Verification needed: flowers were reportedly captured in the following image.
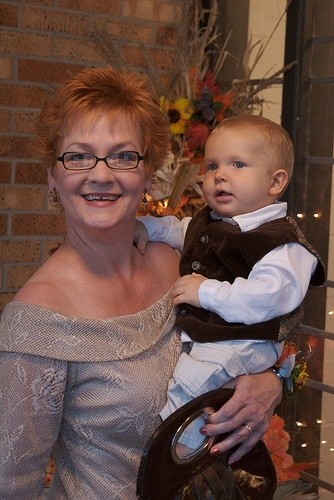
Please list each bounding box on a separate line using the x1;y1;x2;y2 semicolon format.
277;339;315;392
98;3;267;225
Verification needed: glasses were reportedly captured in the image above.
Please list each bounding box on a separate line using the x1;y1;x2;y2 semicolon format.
55;150;146;171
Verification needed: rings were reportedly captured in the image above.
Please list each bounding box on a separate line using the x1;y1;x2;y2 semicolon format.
242;424;253;435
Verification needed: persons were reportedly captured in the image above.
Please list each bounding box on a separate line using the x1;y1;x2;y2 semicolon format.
132;115;328;463
0;65;308;500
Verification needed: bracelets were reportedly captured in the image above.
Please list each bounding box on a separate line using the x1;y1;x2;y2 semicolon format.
269;341;314;396
